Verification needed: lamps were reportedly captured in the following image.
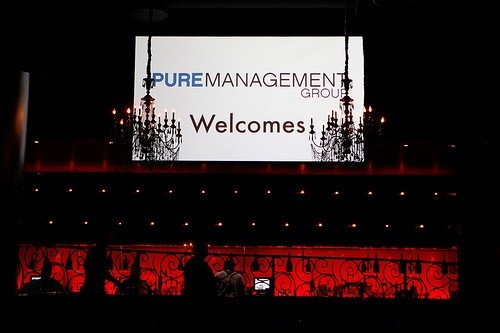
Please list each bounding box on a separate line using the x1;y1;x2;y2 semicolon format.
306;1;388;176
108;1;183;171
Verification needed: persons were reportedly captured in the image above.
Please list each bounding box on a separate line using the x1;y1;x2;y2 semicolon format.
121;265;153;296
214;259;245;297
41;261;64;287
84;236;121;296
183;237;219;297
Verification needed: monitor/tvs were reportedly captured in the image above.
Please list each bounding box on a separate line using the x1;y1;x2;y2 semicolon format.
30;275;41;286
253;276;272;291
